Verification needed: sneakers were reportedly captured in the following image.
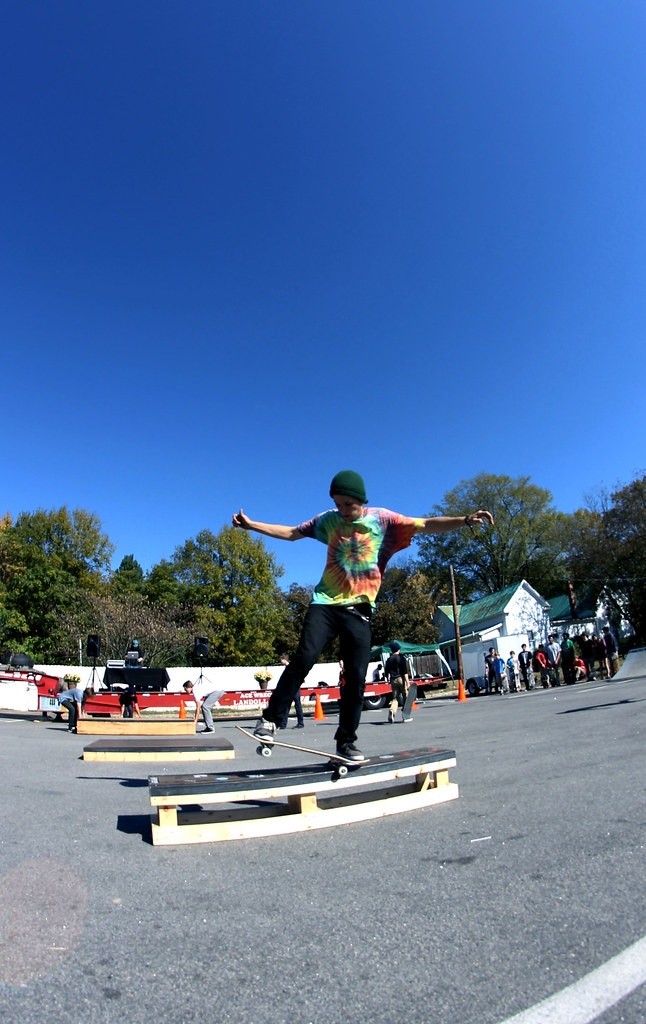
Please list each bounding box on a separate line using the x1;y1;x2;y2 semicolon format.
254;716;275;742
336;739;365;760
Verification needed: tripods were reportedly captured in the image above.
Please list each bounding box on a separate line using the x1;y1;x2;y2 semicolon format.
86;657;103;690
193;660;212;686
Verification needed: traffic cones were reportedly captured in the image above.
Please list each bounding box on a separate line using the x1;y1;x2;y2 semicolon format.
312;693;327;720
455;679;469;701
179;700;187;719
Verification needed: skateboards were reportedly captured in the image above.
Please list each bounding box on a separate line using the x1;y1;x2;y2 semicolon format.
232;723;371;776
514;669;522;691
528;667;536;690
575;678;588;684
546;665;556;687
402;683;418;720
501;671;509;695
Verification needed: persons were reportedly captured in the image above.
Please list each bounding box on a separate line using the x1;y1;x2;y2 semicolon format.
233;470;494;760
59;688;95;732
386;644;413;723
373;664;382;682
281;657;304;729
338;660;345;687
484;627;619;695
183;680;224;734
119;688;142;718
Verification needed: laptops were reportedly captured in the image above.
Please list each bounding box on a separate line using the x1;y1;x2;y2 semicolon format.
128;652;139;659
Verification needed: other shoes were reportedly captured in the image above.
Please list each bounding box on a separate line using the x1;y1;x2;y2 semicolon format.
69;727;75;734
200;729;214;734
403;717;413;722
388;710;395;724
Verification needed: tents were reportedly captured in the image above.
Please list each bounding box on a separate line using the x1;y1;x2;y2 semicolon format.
371;640;454;685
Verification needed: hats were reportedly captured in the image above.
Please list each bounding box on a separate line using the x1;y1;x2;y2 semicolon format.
388;642;400;653
330;471;366;504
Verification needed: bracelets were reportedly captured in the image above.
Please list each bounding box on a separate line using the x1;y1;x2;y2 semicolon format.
465;515;471;527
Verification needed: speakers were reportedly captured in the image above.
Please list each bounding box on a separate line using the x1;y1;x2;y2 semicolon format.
86;634;101;657
194;636;209;659
